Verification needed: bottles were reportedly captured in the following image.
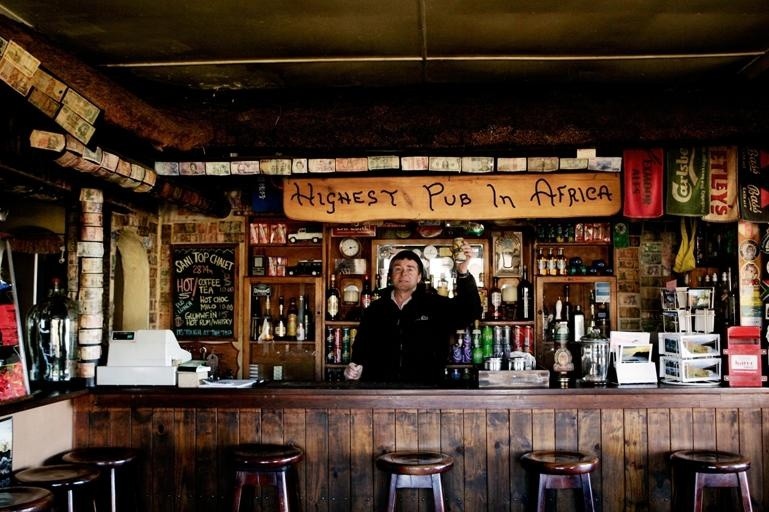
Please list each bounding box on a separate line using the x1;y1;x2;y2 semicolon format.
244;222;740;386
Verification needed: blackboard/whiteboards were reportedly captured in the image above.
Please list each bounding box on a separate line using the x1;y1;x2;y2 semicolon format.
170;243;240;341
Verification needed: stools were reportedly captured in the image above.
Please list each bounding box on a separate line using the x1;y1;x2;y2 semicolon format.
374;450;452;512
0;440;126;511
669;448;754;511
224;441;302;511
518;448;600;511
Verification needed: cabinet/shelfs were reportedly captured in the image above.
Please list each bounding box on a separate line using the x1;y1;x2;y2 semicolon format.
241;217;618;382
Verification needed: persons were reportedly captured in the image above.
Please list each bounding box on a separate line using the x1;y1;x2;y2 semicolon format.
343;242;483;387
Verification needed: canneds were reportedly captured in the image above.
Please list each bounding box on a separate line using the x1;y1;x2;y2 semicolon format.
453;326;536;363
453;237;466;264
326;329;357;365
